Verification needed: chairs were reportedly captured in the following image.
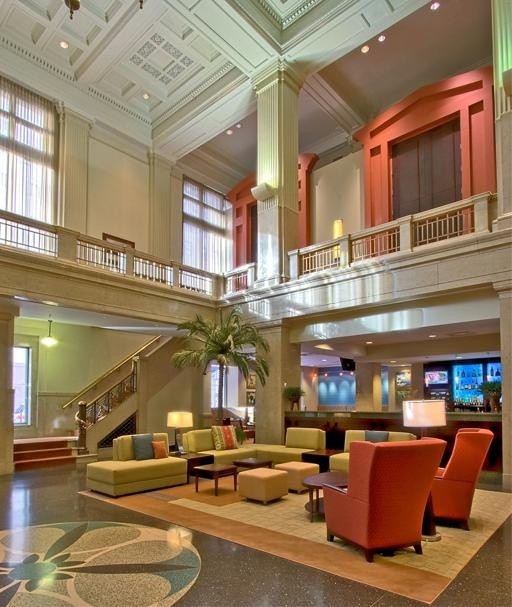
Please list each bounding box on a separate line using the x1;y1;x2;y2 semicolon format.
321;436;448;563
430;428;495;535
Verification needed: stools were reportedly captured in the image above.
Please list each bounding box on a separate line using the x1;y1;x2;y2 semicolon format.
274;460;320;494
236;467;289;504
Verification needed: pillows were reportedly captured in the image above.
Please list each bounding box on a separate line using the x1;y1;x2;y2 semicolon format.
131;432;169;461
211;425;239;450
365;430;390;442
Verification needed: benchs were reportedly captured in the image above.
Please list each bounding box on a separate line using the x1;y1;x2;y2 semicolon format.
257;426;326;469
183;429;283;473
86;431;189;498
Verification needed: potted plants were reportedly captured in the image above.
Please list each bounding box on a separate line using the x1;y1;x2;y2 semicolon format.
477;380;501;413
283;388;306;411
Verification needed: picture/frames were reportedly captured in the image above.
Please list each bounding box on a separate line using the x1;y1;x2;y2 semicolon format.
102;230;136;269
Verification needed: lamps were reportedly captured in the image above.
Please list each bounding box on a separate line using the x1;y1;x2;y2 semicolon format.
402;399;447;440
428;0;440;11
40;313;58;348
167;410;193;454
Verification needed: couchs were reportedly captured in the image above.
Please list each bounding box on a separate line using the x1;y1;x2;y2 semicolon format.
328;428;417;473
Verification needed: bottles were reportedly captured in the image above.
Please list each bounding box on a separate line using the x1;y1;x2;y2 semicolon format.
491;366;501;375
456;367;483;405
